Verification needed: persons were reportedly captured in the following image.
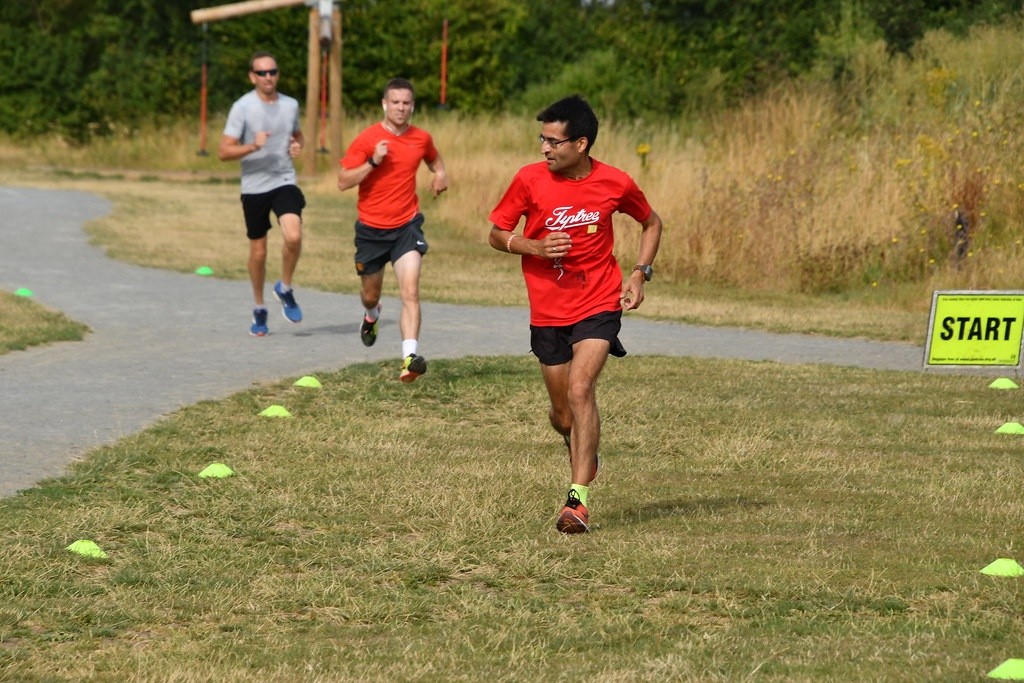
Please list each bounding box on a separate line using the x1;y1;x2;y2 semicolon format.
486;92;662;534
217;53;305;336
335;78;446;384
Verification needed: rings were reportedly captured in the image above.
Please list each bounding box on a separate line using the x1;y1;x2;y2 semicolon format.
553;247;556;252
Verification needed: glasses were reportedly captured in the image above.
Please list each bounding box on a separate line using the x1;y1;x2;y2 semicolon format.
252;68;277;76
539;132;581;148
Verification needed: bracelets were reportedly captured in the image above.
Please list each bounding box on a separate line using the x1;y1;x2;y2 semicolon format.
368;156;379;168
507;235;516;253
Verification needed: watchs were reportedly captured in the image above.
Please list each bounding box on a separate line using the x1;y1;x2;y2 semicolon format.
632;264;653;281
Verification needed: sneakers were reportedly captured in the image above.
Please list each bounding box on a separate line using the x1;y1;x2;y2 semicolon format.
250;304;270;337
400;354;426;384
274;281;303;324
555;488;588;533
359;302;383;347
